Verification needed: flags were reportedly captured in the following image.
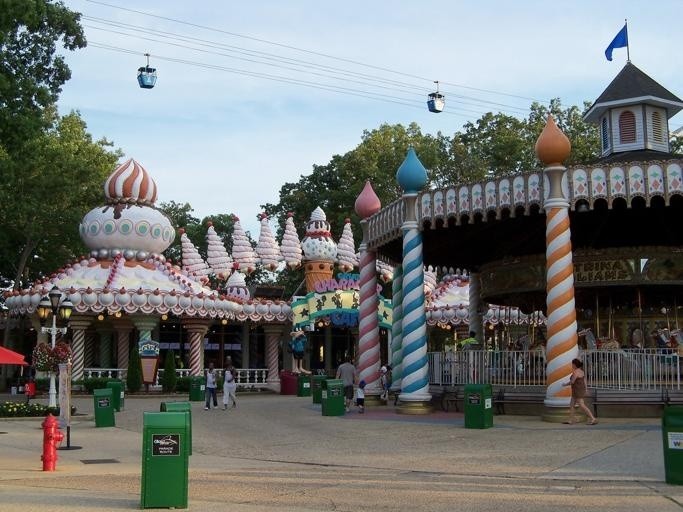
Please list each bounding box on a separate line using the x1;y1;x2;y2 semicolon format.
605;24;628;61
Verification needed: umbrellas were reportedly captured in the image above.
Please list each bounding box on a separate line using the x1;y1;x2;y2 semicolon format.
0;347;29;366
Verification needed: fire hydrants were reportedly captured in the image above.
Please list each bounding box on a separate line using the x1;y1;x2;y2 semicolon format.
38;413;64;470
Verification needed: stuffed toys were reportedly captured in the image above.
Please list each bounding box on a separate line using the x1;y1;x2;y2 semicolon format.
288;322;312;375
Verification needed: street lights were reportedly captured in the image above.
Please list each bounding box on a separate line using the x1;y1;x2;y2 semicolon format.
36;285;73;407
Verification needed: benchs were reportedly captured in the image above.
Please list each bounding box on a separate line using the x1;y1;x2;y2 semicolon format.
445;386;500;413
391;385;445;411
496;388;545;414
663;389;683;406
0;393;29;403
591;387;666;418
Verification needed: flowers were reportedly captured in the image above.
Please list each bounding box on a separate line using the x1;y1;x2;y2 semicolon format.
31;342;74;371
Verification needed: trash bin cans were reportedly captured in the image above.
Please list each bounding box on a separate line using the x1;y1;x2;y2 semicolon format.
313;375;327;403
465;384;493;429
662;406;683;485
322;379;344;416
189;377;205;401
107;382;124;412
141;402;192;509
297;376;311;397
93;388;115;427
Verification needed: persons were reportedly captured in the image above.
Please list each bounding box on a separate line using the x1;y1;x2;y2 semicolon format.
355;381;367;413
174;354;182;376
351;357;356;367
204;361;219;410
561;359;599;425
456;331;481;349
335;355;358;413
380;360;393;402
184;351;190;369
336;357;342;369
221;358;238;411
